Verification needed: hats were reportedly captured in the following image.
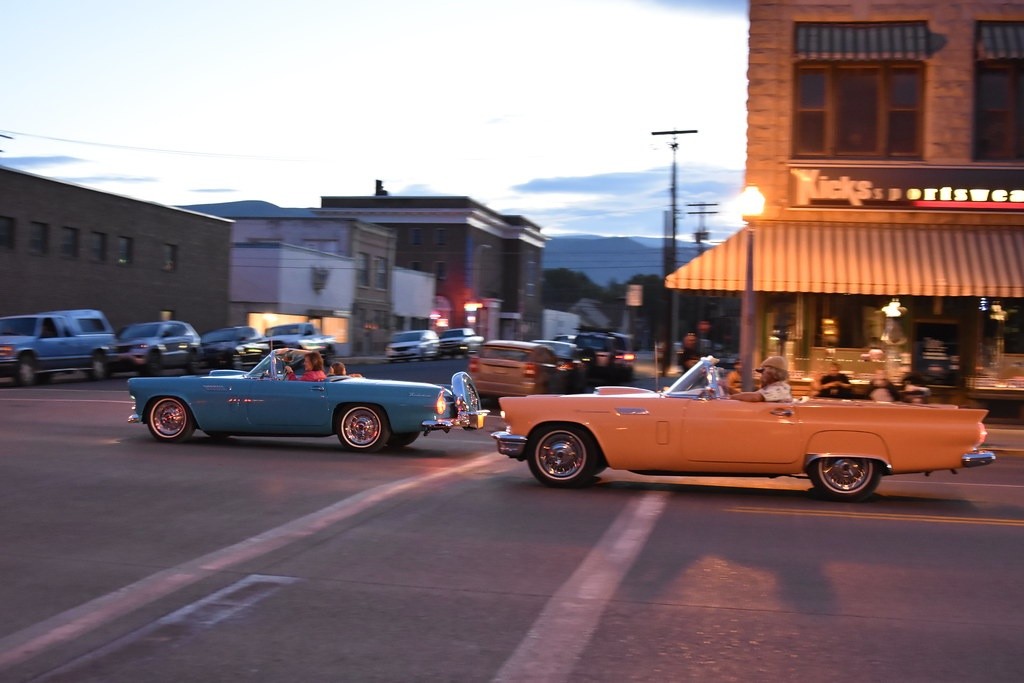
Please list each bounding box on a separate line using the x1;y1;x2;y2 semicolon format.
872;369;888;380
760;355;788;371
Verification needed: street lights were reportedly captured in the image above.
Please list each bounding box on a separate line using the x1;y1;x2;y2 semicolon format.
651;129;700;377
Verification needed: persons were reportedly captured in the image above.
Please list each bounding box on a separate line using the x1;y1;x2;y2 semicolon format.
694;318;715;357
717;355;793;403
864;369;901;403
329;362;346;375
816;362;853;399
283;351;327;382
678;331;701;376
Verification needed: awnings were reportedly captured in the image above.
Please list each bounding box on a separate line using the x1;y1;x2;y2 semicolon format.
666;223;1024;298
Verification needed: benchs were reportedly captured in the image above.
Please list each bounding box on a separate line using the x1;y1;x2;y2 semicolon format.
319;376;350;382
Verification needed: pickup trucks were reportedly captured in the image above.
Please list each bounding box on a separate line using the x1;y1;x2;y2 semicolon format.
1;309;120;388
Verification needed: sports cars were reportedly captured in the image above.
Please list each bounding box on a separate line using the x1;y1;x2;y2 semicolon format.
491;356;996;502
126;348;492;452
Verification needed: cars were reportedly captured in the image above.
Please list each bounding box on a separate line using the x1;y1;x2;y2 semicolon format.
469;341;574;401
554;331;639;381
263;324;336;367
111;321;204;377
436;327;485;358
385;329;441;363
200;326;270;370
533;339;591;394
